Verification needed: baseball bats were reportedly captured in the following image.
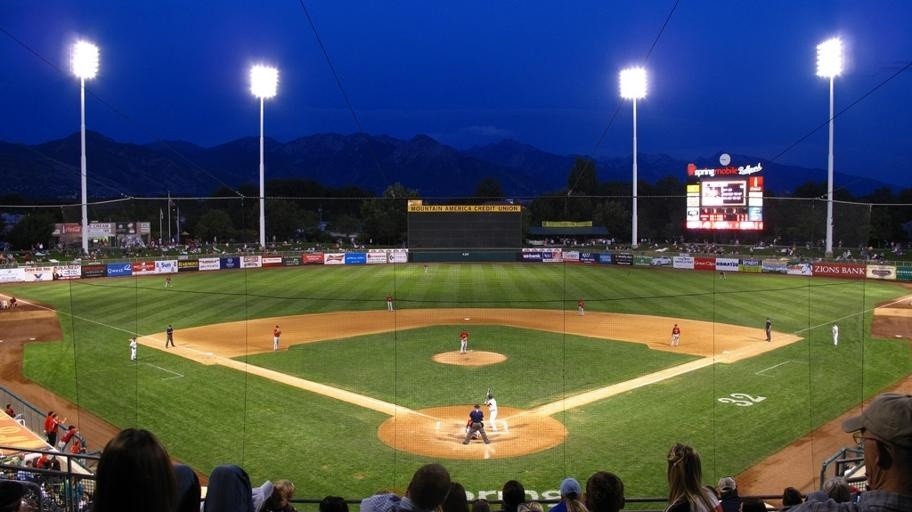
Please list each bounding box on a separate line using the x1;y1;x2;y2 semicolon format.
483;388;490;405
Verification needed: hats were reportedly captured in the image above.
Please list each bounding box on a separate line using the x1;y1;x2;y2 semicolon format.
842;392;912;447
718;477;737;493
824;477;850;502
252;480;273;512
561;478;580;499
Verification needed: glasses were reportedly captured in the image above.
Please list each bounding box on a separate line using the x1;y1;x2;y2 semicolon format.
853;432;889;448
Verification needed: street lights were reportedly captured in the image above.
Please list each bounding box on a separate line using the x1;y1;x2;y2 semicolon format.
248;62;280;252
619;65;650;251
69;40;102;256
816;35;850;263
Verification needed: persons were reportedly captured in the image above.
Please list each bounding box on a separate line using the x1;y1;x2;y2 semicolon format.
831;322;838;346
459;329;469;354
665;443;767;511
578;295;585;316
319;496;349;511
9;296;16;311
385;294;394;312
719;270;727;280
273;325;281;352
782;476;862;508
165;275;173;288
44;411;81;457
543;235;912;266
84;427;295;511
0;238;367;269
129;337;137;361
462;404;490;444
2;446;84;511
358;464;626;512
6;404;16;418
485;395;498;432
165;324;176;349
52;267;62;281
797;396;912;510
764;317;771;342
671;324;681;347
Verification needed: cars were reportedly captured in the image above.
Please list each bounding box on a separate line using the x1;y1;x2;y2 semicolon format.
650;255;671;267
